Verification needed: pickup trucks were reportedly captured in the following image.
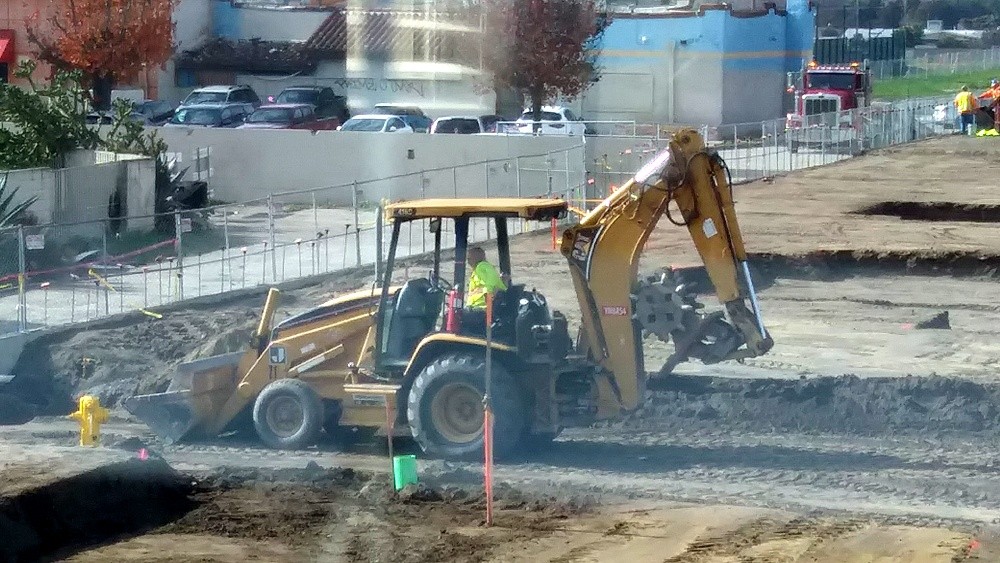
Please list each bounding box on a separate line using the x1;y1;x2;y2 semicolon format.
233;102;338;131
274;84;348;118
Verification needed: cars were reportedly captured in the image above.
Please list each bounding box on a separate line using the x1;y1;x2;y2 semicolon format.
127;98;175;127
84;110;157;127
429;115;509;134
515;105;588;135
369;102;434;134
163;100;248;128
336;113;414;132
932;105;960;129
173;84;262;124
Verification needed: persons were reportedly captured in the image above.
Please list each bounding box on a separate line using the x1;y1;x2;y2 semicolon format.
955;85;976;134
459;247;507;332
978;80;1000;132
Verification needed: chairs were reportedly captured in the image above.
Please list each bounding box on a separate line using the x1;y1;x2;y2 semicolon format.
467;287;514;334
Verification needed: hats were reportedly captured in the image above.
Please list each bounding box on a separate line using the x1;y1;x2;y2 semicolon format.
990;78;1000;87
961;85;969;92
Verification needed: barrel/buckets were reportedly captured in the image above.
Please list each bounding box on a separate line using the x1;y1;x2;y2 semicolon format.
393;454;418;491
967;123;977;136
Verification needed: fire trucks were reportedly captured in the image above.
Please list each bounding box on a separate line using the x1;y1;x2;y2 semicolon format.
782;59;895;156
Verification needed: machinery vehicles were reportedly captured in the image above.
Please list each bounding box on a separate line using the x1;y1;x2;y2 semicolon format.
119;128;779;462
